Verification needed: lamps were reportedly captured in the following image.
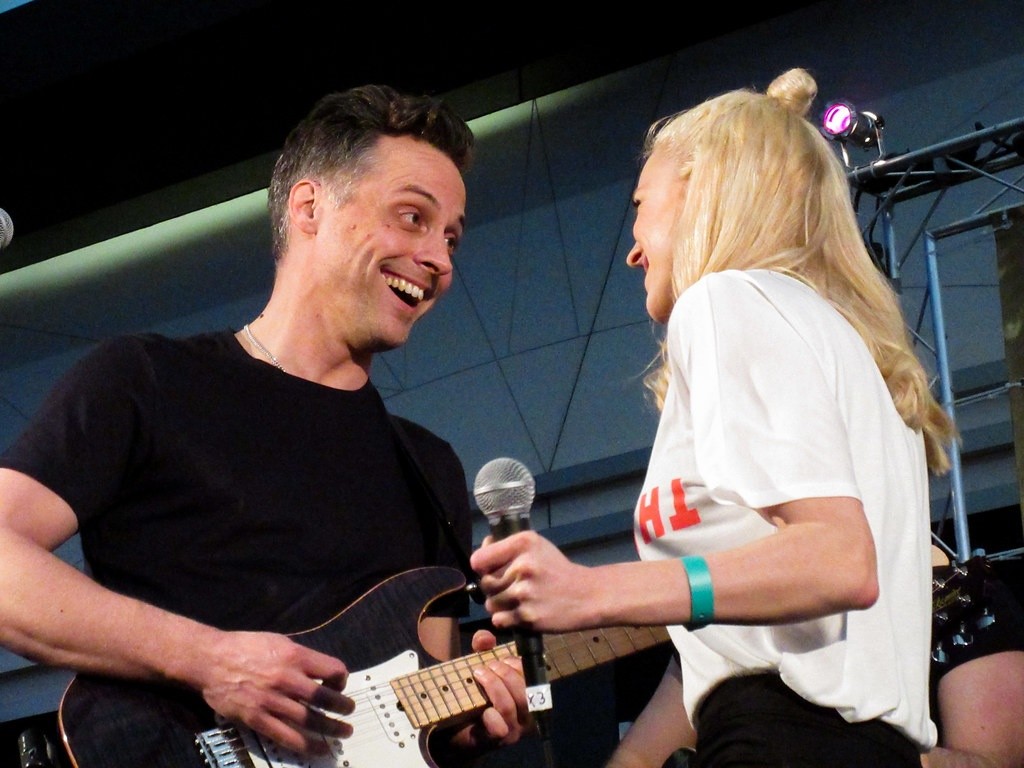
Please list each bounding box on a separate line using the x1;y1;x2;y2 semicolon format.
818;98;886;169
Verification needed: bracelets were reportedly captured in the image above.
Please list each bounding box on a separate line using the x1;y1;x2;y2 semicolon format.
681;556;713;632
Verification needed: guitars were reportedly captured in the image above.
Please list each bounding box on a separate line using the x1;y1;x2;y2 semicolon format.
54;547;1000;768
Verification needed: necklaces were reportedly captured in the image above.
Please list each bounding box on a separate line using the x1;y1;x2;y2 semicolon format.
244;324;286;372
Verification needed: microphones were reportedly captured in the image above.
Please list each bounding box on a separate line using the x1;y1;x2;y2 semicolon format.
474;457;553;742
0;208;14;250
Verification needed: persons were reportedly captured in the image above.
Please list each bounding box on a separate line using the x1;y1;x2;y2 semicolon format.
469;68;956;768
0;89;540;768
603;547;1024;768
